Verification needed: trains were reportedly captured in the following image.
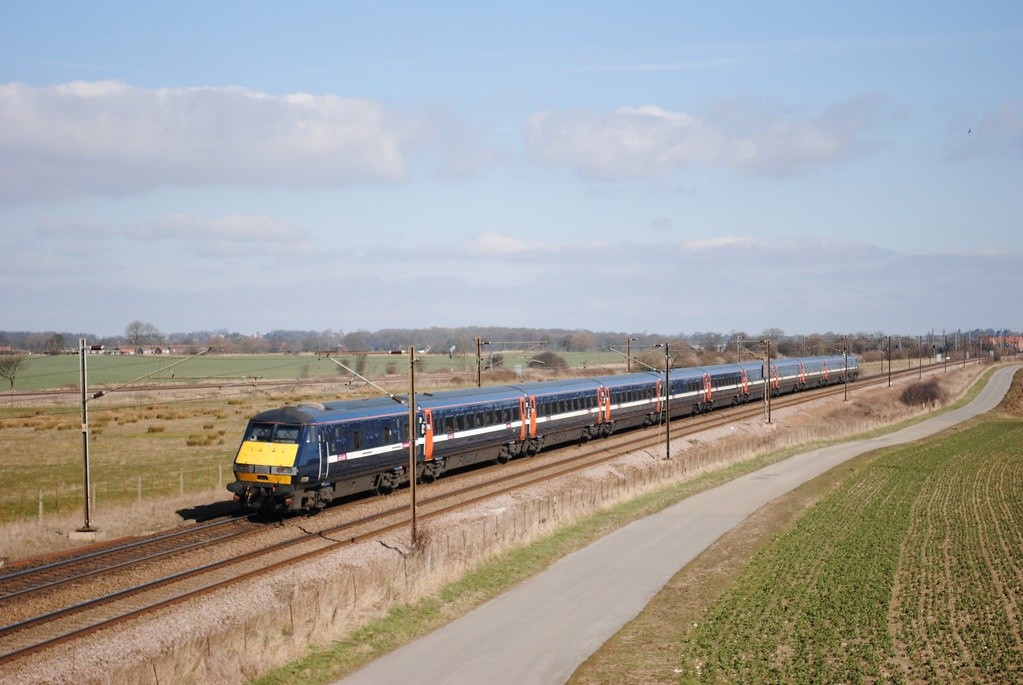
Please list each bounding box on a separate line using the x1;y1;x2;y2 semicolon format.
222;354;859;523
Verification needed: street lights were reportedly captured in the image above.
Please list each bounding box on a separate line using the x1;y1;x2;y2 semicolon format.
736;330;1023;376
623;336;641;372
723;338;774;424
320;342;424;542
78;338;222;532
840;334;852;402
882;334;893;388
471;336;552;389
600;342;685;460
